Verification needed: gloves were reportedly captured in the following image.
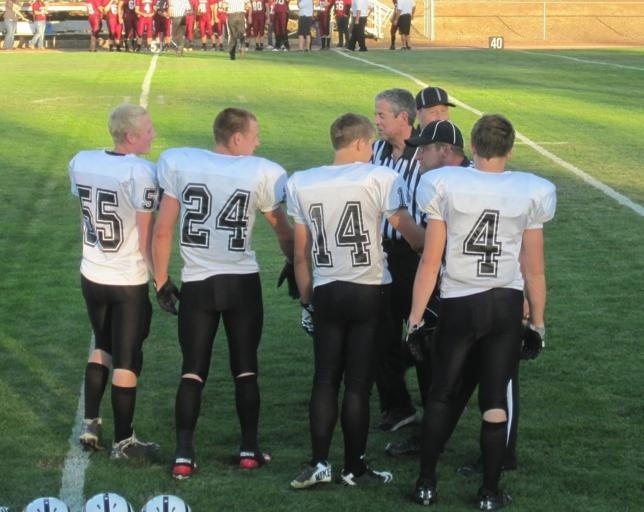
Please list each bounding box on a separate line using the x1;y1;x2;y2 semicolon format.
521;321;545;360
300;303;314;336
157;274;180;315
277;258;301;299
405;320;428;362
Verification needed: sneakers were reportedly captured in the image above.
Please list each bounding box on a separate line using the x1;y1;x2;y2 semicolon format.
376;407;416;431
295;43;368;53
244;44;290;52
171;459;197;480
390;45;411;51
385;440;421;457
476;486;512;512
89;44;225;52
291;461;332;489
111;427;160;461
414;480;438;505
78;418;106;450
458;459;484;475
239;449;271;470
339;468;393;488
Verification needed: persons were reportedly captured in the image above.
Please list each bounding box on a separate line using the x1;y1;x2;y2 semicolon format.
87;0;375;59
415;84;457;129
390;0;417;51
285;113;427;495
150;103;296;479
67;102;159;465
378;119;534;475
364;88;424;434
2;0;23;50
30;0;48;49
401;112;557;511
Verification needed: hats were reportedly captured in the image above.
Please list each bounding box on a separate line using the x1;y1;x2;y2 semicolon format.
404;120;465;149
416;87;456;109
